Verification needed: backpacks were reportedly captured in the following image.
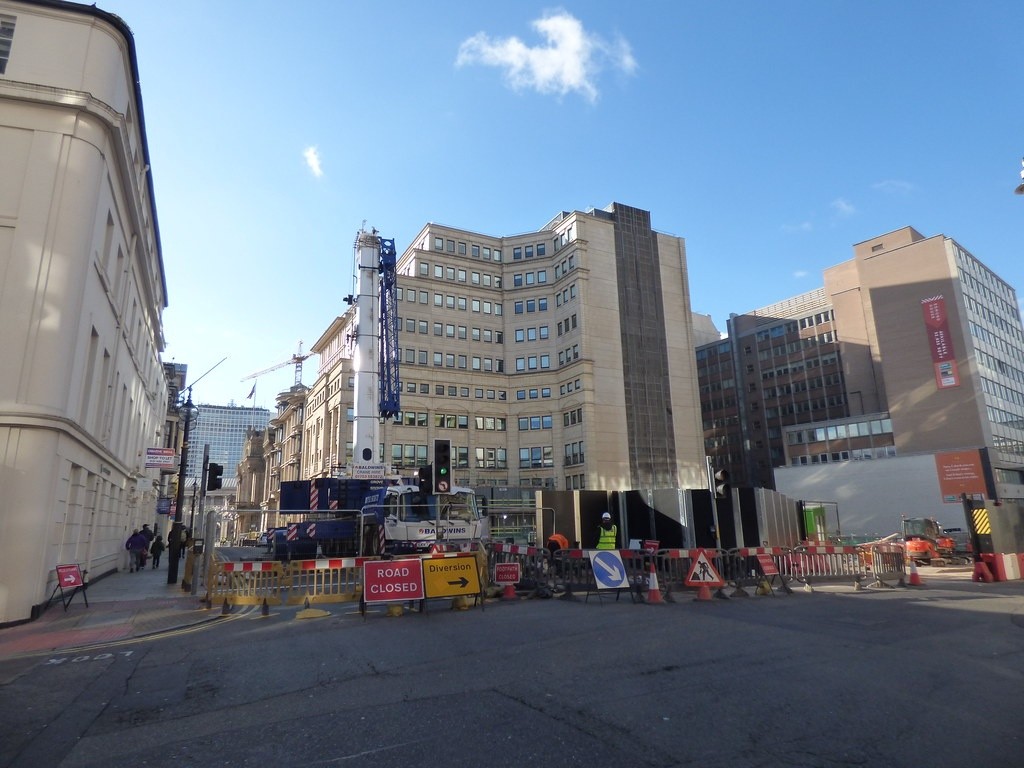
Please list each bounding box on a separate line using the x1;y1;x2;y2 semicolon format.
181;529;187;542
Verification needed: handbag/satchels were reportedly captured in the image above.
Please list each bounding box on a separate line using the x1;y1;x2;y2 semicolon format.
141;549;148;560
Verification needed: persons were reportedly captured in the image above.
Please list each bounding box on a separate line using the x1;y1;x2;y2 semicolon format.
125;523;189;573
592;512;622;550
547;534;568;577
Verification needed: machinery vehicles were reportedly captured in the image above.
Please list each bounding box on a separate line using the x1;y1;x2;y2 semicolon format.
904;516;957;563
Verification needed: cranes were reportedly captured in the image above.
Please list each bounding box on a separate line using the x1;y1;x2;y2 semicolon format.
242;338;314;390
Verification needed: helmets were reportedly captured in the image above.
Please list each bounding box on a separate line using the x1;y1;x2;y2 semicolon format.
602;512;611;520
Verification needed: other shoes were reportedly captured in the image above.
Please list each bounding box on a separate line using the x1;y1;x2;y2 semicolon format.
130;568;133;573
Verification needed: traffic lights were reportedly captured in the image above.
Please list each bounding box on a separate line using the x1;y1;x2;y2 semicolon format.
712;469;731;500
207;463;224;491
434;438;452;496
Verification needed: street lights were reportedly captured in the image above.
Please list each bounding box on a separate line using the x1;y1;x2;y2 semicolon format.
167;383;199;583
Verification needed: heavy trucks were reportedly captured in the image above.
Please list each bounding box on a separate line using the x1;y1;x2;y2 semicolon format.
271;484;482;558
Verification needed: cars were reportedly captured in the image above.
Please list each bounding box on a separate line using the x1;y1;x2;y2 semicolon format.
238;531;268;547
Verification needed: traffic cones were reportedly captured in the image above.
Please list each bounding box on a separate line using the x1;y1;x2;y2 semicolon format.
908;553;926;587
644;561;668;604
501;584;520;601
693;585;718;602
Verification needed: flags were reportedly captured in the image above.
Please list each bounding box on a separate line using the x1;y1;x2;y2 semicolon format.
247;385;255;400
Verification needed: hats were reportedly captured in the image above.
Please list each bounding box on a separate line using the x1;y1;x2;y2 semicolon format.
143;523;151;528
